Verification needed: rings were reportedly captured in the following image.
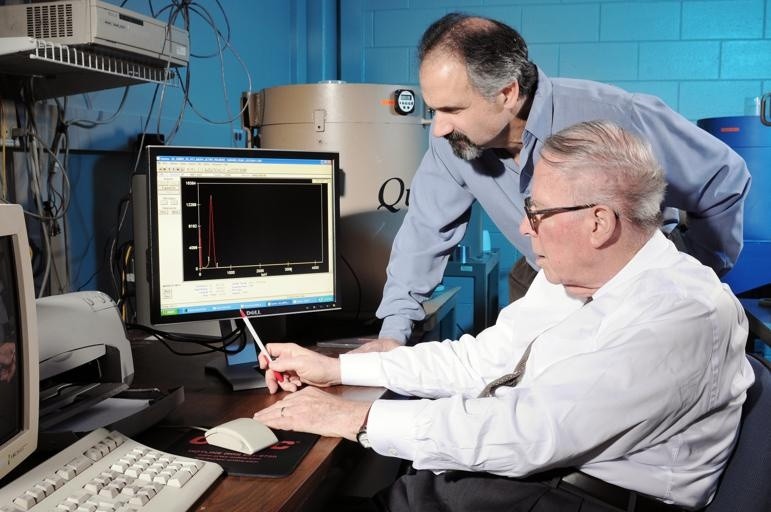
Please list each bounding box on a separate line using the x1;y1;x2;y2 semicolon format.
277;406;286;417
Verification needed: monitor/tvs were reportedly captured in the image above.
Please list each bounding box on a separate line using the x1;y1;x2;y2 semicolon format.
0;203;40;482
145;144;343;391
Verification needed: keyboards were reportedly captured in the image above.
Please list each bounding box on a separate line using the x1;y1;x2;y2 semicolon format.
0;426;225;512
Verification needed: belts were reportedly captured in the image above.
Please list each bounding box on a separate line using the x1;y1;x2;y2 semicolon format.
555;463;680;511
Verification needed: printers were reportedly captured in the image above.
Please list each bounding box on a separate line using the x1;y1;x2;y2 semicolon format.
36;290;185;445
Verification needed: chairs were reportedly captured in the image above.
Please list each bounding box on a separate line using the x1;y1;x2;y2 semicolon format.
707;352;771;512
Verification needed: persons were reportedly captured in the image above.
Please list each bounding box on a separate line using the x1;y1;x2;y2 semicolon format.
251;118;755;511
342;13;752;353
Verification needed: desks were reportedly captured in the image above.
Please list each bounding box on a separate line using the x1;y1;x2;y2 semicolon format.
0;345;405;512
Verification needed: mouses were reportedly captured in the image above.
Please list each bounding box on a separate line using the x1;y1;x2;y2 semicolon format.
203;417;279;455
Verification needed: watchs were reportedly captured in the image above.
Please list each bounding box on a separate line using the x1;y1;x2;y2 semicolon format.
359;404;375;452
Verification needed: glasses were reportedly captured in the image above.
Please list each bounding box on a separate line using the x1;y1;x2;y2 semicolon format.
523;196;619;235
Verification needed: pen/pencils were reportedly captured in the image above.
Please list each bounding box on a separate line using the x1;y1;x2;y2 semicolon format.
240;309;283;382
